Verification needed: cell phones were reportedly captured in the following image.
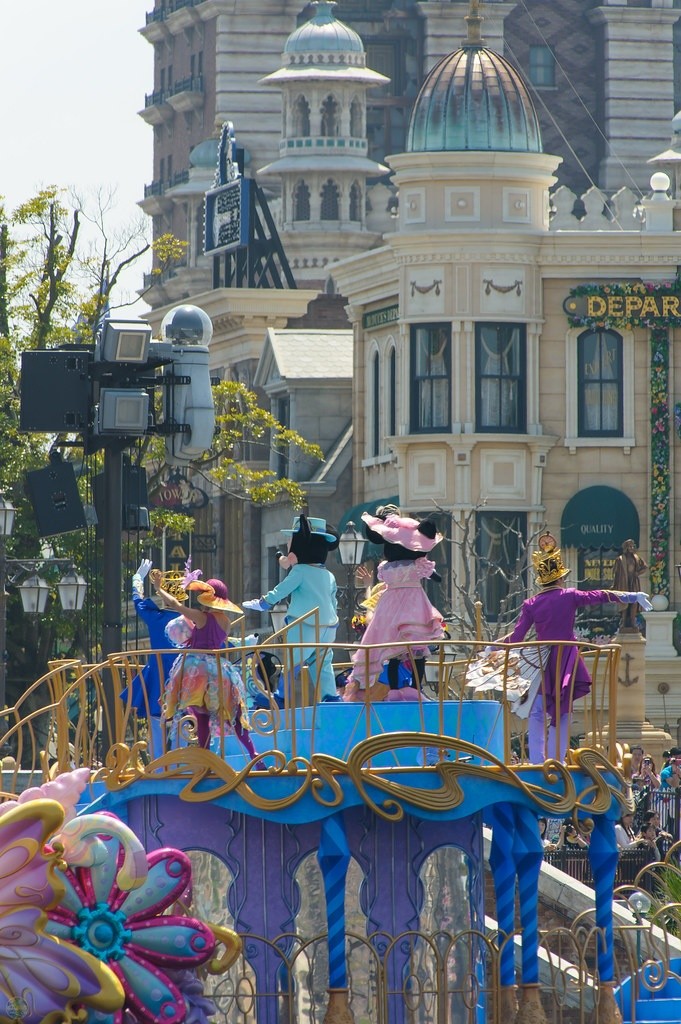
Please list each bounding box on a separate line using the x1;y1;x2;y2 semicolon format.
670;758;676;762
645;759;649;765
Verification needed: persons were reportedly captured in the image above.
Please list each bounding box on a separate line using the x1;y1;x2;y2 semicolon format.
120;559;268;771
507;745;681;900
488;547;653;765
355;565;373;622
613;539;648;632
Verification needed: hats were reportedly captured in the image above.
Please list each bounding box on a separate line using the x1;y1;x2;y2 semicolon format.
185;578;244;615
530;545;572;586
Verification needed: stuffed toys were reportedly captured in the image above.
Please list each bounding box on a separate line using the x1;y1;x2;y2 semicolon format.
242;512;445;709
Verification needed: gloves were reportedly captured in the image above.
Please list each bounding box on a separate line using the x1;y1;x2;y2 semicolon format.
135;559;153;579
636;592;653;612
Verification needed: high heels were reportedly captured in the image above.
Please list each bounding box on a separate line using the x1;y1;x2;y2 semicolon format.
251;752;267;770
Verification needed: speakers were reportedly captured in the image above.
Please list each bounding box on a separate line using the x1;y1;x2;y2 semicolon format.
90;466;150;540
26;462;87;539
20;350;94;432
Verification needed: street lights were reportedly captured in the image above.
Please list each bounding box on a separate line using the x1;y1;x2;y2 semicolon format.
266;520;383;662
0;490;92;761
87;317;157;767
